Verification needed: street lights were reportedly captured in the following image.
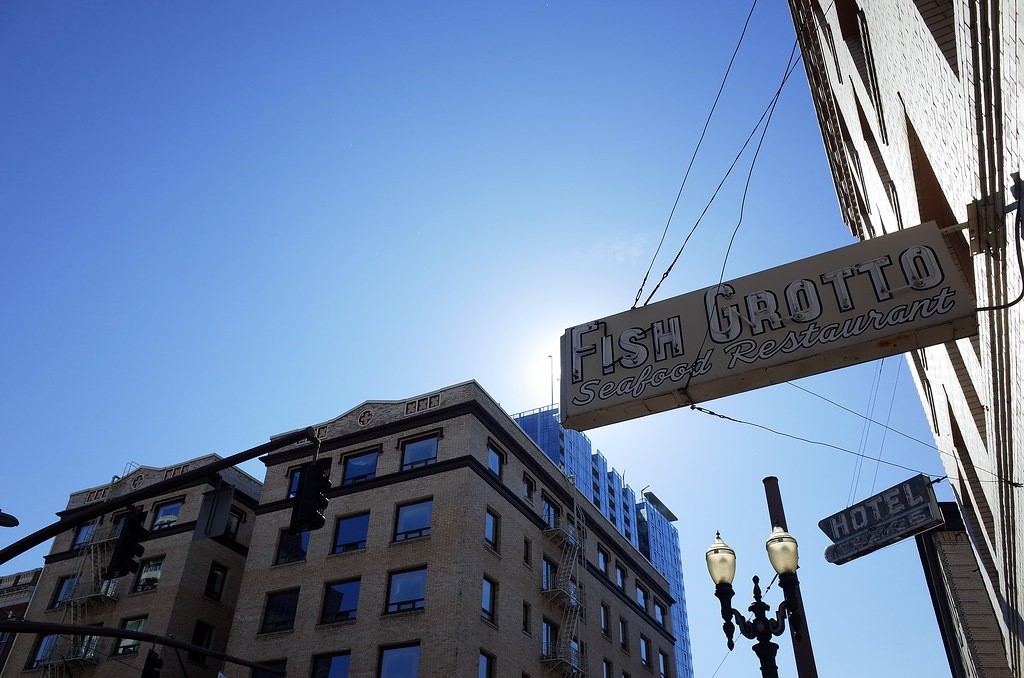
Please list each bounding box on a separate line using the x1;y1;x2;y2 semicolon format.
705;521;802;678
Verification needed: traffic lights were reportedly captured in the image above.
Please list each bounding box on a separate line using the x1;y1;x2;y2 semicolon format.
101;510;151;582
289;460;332;531
